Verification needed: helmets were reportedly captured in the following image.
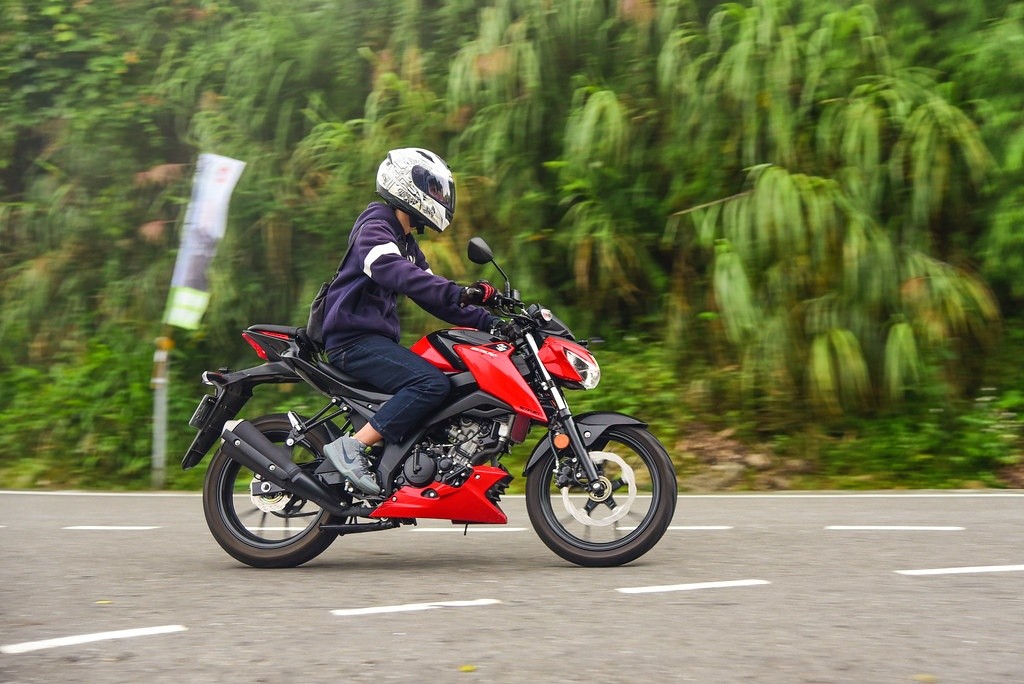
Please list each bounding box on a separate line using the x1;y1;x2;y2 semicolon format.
374;145;457;234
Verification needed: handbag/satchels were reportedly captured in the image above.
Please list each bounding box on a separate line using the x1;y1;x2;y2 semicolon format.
305;280;329;348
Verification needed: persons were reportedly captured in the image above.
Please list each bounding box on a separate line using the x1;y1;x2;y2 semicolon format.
306;146;496;498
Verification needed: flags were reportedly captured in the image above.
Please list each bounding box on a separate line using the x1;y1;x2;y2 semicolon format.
160;152;248;332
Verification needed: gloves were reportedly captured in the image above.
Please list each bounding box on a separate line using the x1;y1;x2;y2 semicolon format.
461;280;496;306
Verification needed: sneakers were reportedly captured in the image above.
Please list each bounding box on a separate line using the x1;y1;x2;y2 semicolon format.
322;431;381;496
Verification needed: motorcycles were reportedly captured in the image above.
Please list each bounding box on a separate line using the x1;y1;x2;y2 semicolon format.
182;236;680;569
